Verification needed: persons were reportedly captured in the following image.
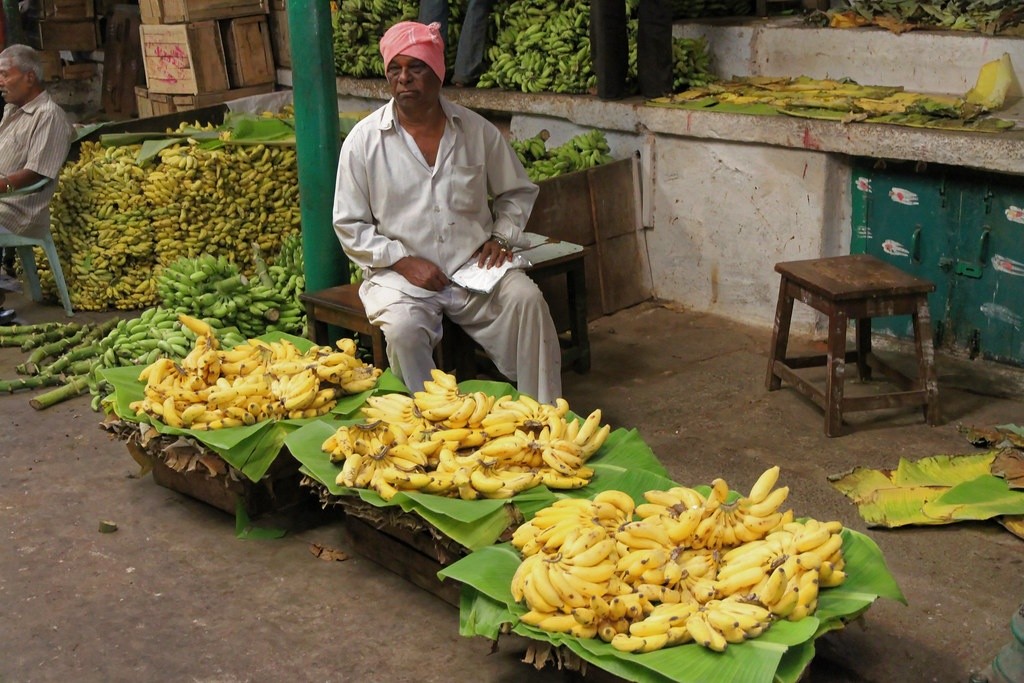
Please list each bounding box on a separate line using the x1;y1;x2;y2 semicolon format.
331;20;564;401
0;44;71;326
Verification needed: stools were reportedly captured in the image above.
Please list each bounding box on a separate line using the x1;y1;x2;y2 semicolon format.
466;230;590;388
764;253;947;439
298;278;466;383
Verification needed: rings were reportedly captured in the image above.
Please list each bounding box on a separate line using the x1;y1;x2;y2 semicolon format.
501;248;508;252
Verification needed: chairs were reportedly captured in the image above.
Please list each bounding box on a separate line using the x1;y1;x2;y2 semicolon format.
0;178;76;319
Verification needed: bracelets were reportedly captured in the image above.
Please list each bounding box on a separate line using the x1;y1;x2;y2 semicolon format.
2;177;14;191
491;236;511;249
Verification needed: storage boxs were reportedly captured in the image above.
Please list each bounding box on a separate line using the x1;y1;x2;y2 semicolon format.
20;0;292;119
97;330;907;683
